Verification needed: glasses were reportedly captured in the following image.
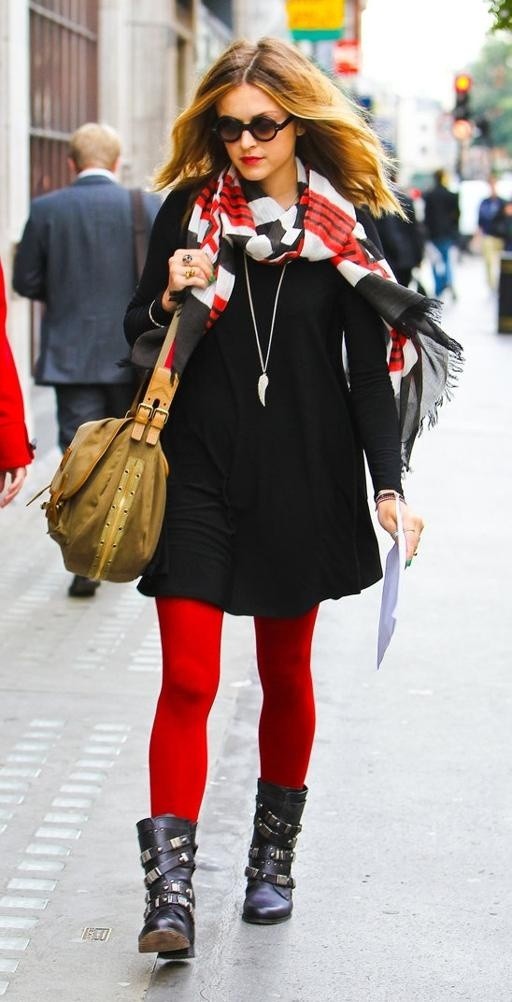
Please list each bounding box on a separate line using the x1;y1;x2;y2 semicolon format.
206;113;297;144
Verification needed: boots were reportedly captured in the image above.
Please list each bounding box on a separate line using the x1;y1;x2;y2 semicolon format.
134;817;195;961
243;777;307;926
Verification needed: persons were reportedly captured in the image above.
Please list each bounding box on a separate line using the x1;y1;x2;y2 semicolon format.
10;121;162;597
122;35;425;958
419;169;460;302
373;174;424;286
478;174;510;297
1;261;35;510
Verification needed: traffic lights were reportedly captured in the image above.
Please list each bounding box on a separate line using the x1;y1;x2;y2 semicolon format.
448;76;475;144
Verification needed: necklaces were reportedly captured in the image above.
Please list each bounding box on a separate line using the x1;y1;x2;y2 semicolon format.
238;250;288;410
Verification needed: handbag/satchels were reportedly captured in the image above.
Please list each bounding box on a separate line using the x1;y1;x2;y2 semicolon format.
44;417;171;581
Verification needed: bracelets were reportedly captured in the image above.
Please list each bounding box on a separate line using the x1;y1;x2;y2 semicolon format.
373;487;405;507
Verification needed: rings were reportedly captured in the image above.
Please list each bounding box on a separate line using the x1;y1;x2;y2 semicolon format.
391;529;399;540
402;528;415;534
182;255;192;266
186;269;194;278
